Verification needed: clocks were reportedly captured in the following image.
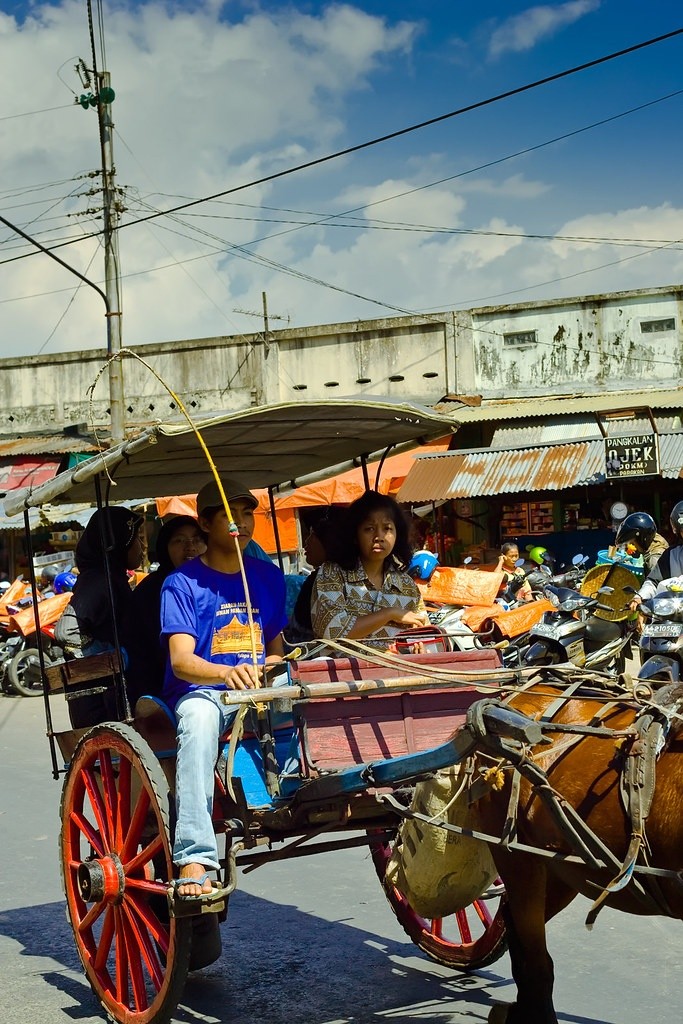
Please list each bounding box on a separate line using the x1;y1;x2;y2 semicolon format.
609;503;628;521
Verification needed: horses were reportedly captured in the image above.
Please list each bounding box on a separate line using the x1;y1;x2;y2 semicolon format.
466;683;683;1024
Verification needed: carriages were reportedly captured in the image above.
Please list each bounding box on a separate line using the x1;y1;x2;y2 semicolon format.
3;393;683;1024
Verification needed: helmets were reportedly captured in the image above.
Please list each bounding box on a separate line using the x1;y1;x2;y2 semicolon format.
670;501;683;534
41;565;61;588
53;572;77;594
615;512;657;554
411;548;440;578
530;547;547;565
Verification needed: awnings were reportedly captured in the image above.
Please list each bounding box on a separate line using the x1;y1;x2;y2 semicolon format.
0;435;110;457
394;434;683;504
0;497;154;530
156;434;452;557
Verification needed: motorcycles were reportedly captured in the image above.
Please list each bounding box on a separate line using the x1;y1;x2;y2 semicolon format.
1;541;683;698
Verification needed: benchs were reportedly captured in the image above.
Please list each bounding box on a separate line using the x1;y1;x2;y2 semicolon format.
136;693;292;740
45;649;176;761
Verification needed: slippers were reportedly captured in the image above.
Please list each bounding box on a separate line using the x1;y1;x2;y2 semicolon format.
177;873;216;900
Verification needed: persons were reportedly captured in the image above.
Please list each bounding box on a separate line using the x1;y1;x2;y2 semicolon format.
494;501;683;754
54;490;431;902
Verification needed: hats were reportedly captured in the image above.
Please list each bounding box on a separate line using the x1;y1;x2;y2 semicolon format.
196;479;258;514
